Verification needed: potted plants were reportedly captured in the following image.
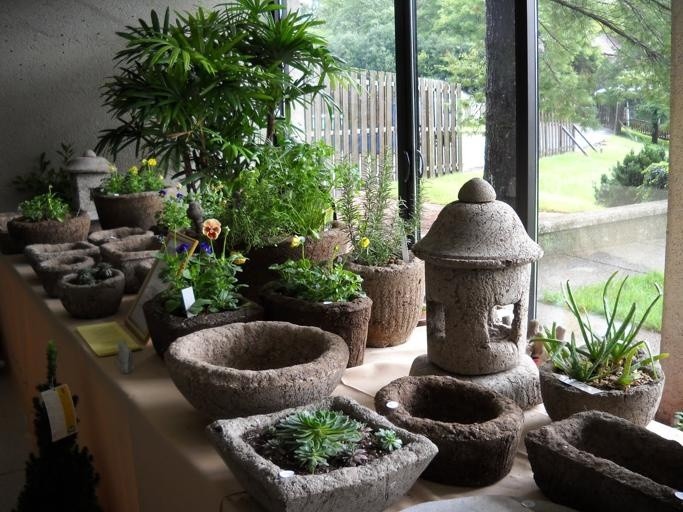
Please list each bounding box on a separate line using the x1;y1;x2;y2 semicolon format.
8;187;90;251
239;140;348;279
279;407;401;466
529;269;671;427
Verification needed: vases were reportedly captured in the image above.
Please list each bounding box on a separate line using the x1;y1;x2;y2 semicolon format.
375;373;523;489
28;240;98;283
102;236;170;289
93;189;169;231
61;264;127;320
527;411;683;512
209;396;438;511
288;293;370;367
92;226;156;253
144;292;263;364
39;254;95;298
168;321;348;426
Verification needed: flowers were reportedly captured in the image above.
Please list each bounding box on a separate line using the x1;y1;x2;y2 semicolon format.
97;159;170;197
166;216;249;316
335;148;422;349
270;228;370;302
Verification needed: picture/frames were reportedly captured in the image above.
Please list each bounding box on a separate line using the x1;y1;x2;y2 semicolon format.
124;234;199;340
